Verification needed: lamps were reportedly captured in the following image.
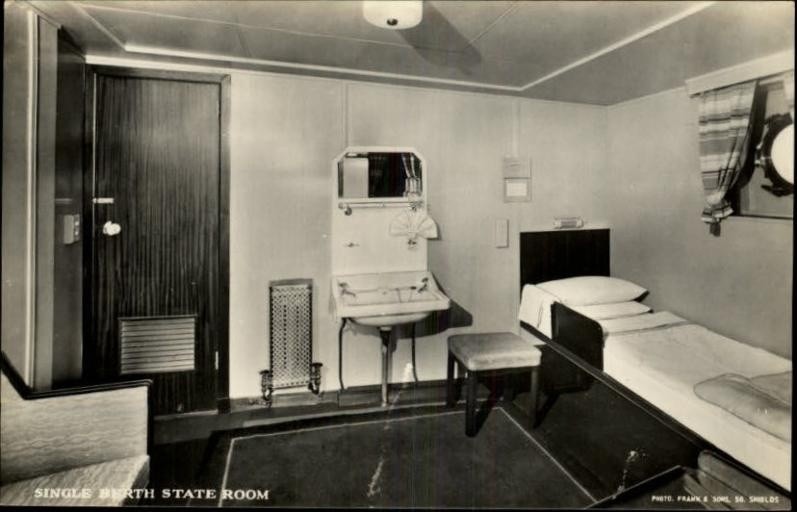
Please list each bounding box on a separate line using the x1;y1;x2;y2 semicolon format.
362;0;424;29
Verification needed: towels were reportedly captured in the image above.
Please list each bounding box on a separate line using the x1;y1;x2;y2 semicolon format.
518;280;562;339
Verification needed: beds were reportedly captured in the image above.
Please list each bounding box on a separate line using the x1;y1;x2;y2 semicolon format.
517;228;793;512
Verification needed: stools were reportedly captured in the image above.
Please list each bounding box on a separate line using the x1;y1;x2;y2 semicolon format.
443;332;541;437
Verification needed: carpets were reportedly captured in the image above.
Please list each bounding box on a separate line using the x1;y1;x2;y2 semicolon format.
184;396;614;508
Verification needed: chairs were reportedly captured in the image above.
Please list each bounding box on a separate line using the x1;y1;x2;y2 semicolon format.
1;350;154;507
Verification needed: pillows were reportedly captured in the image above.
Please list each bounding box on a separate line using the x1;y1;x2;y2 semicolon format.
566;299;652;319
535;275;648;305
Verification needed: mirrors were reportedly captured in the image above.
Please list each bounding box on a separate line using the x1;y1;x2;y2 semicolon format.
335;146;424;199
